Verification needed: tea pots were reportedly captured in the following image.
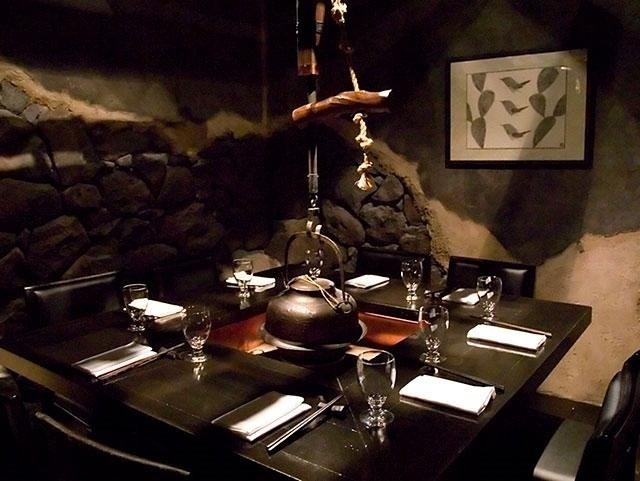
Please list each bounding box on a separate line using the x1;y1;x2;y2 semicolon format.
265;230;362;349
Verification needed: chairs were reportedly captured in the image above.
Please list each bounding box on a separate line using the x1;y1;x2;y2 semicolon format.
22;269;126;331
444;255;539;299
352;244;437;293
468;350;640;480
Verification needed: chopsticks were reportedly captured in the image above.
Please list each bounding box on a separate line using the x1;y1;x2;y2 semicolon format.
265;390;352;455
422;360;507;394
492;319;553;337
96;340;185;383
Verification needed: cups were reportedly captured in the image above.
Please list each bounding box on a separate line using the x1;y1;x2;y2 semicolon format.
417;305;450;363
121;283;149;333
400;260;423;302
232;258;254;300
475;274;503;323
180;305;212;363
356;349;397;427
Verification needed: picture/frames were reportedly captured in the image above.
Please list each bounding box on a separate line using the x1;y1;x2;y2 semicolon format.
445;46;593;170
